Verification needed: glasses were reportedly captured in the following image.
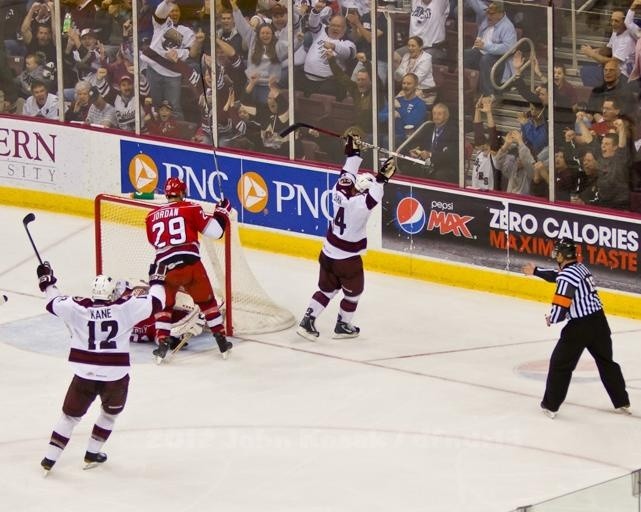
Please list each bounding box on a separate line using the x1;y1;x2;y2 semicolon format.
552;249;559;259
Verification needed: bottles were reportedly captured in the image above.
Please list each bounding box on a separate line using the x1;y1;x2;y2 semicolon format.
62;10;72;34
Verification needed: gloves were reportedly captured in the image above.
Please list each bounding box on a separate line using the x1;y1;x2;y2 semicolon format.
380;158;396;179
214;198;231;217
37;261;57;291
345;134;361;155
149;264;167;284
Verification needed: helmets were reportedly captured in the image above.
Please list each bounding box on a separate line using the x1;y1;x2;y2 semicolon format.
551;237;578;260
92;274;117;301
354;172;376;192
164;177;187;196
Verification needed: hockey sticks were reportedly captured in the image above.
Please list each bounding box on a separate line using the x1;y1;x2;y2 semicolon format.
23;213;43;264
280;123;427;165
200;51;225;203
162;294;224;361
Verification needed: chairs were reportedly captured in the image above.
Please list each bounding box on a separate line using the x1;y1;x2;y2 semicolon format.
573;86;594;103
630;192;641;214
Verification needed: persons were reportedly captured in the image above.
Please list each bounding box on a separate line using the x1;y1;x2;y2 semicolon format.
144;176;233;359
523;237;635;417
120;278;205;351
36;261;169;469
297;133;398;343
1;0;640;214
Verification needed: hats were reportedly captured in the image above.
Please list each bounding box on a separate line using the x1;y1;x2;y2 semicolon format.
79;28;97;38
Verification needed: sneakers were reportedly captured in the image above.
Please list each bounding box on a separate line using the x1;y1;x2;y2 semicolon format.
299;308;319;337
540;402;559;412
333;314;360;334
214;335;232;353
153;339;168;359
84;451;107;462
40;457;56;470
